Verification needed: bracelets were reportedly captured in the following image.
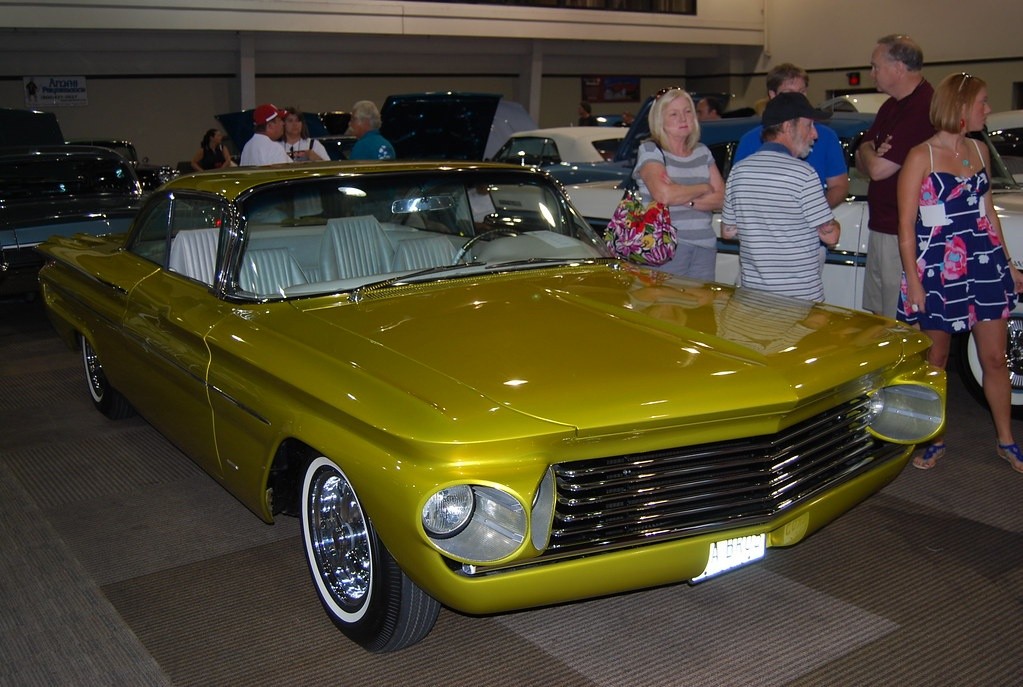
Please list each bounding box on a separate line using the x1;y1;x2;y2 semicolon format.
1006;257;1012;262
689;200;694;208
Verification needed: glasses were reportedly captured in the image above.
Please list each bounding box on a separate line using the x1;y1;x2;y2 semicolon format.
958;72;972;92
872;60;899;72
656;86;683;102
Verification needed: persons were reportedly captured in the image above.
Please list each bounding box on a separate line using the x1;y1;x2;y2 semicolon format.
623;111;635;126
625;271;714;328
192;129;231;171
630;86;726;283
857;34;936;319
735;62;849;209
696;96;722;120
578;101;598;126
721;92;840;303
240;103;331;166
713;288;829;356
349;101;396;160
895;73;1023;474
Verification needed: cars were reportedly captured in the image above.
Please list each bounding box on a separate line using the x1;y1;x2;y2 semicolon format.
37;163;947;654
213;93;1023;421
0;107;180;299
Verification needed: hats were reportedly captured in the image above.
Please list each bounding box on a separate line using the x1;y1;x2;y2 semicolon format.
762;93;832;130
253;104;289;126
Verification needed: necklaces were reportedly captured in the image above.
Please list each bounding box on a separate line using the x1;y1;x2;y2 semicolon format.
952;153;976;173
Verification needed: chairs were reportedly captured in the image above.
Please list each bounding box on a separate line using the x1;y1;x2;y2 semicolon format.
238;246;310;296
319;215;395;282
75;171;99;190
168;228;220;286
991;156;1023;183
848;167;871;196
391;235;465;272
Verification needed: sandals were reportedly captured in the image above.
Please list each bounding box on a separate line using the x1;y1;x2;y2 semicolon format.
996;440;1023;473
912;443;945;469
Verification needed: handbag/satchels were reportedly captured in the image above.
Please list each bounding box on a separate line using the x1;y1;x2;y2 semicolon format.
602;140;678;268
230;161;238;167
